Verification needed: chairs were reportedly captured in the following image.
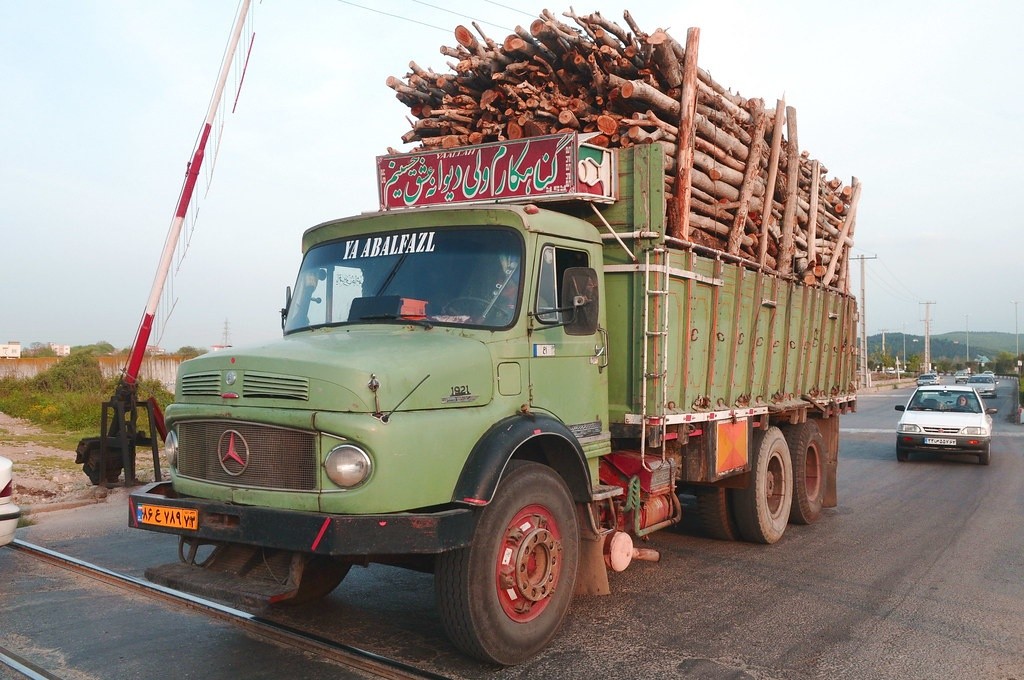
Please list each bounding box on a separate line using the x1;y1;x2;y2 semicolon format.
922;399;939;410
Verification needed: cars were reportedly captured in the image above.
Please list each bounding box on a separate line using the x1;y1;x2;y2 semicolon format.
886;367;905;374
963;370;999;399
917;373;941;386
0;454;20;548
894;386;997;465
954;371;969;384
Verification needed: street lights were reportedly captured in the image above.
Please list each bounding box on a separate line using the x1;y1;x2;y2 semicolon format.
962;311;973;361
899;321;909;365
1010;298;1023;357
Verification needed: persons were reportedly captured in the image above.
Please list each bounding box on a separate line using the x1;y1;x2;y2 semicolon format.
956;395;967;407
492;267;548;323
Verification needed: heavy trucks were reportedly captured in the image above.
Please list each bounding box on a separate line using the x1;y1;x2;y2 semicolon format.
128;133;862;666
